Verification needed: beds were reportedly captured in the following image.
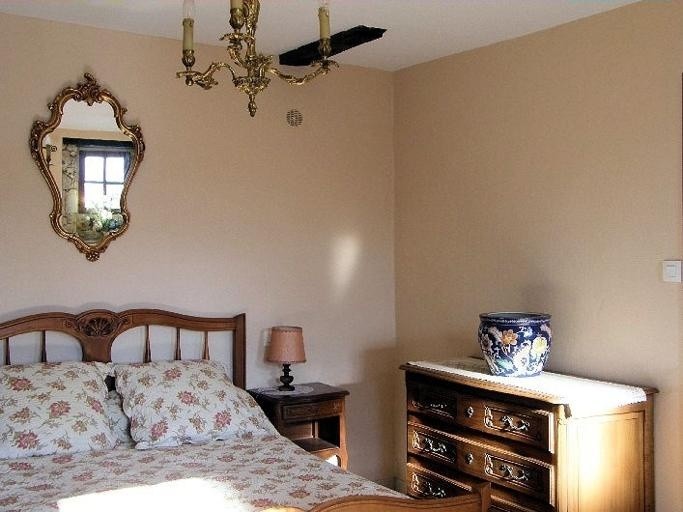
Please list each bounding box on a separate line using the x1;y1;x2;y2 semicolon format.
0;308;493;511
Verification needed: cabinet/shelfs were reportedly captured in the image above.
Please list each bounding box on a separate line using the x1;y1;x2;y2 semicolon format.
398;356;659;512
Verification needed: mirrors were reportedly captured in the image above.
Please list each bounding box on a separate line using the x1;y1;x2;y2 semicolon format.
29;72;145;261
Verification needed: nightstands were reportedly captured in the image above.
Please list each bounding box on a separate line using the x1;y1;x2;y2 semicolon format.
248;382;349;470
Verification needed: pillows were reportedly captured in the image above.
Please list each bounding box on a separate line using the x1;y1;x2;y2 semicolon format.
0;360;119;457
113;361;238;451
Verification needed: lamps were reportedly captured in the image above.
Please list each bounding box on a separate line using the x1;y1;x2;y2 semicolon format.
173;0;338;116
266;325;307;391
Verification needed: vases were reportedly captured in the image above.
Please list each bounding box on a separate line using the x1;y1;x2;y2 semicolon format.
478;312;553;377
